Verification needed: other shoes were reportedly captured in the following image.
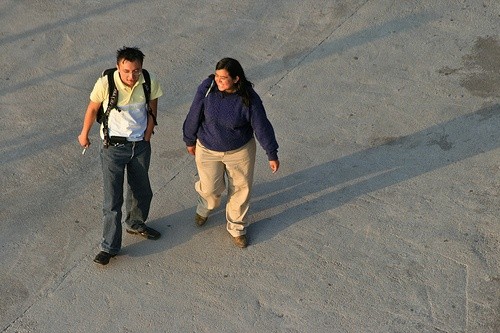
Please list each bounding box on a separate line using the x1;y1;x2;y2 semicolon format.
196;212;208;225
93;251;115;266
234;235;247;248
126;224;160;240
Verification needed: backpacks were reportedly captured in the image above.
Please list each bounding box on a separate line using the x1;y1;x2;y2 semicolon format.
95;68;151;125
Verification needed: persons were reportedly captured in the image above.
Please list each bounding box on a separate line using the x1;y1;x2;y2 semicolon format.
183;57;280;248
77;44;163;265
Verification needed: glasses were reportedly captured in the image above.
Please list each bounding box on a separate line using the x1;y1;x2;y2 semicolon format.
123;69;142;76
